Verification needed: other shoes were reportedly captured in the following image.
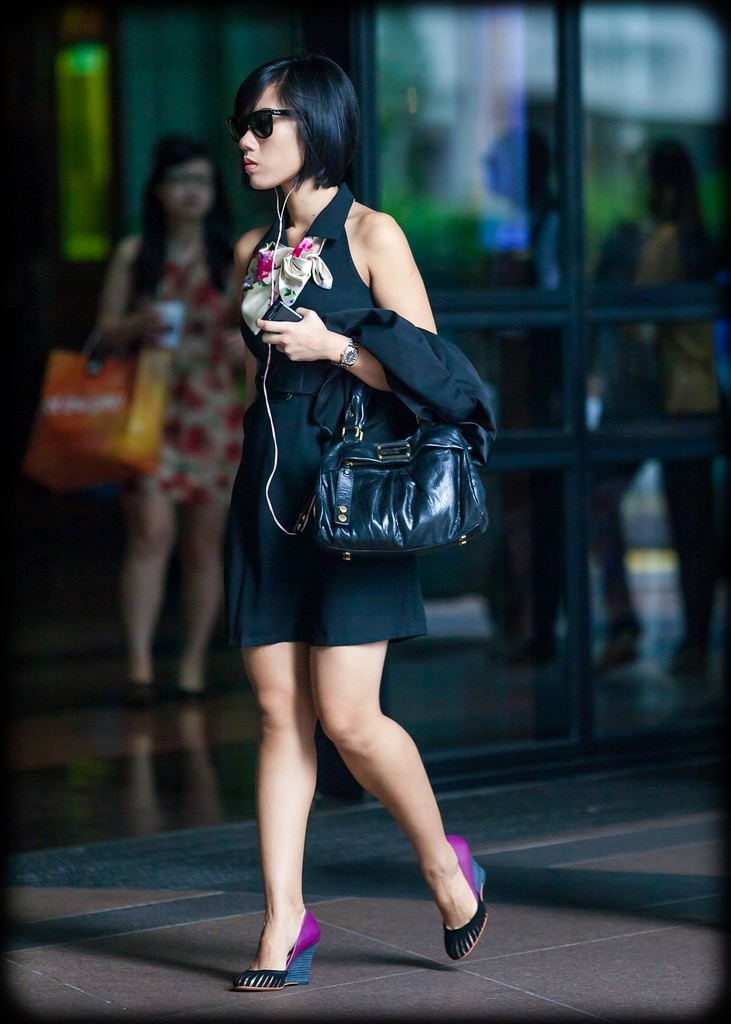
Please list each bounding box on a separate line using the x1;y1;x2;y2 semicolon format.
669;641;706;682
129;671;156;703
175;671;205;703
597;614;640;669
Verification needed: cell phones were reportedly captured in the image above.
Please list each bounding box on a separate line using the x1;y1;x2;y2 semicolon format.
262;300;303;323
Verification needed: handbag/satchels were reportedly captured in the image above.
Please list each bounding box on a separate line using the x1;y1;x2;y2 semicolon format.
307;365;487;561
23;320;165;493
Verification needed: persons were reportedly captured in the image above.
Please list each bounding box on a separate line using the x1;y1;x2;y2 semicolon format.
93;134;245;701
489;127;716;674
221;55;485;993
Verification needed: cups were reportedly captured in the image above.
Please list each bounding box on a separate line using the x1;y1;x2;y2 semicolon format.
150;302;183;349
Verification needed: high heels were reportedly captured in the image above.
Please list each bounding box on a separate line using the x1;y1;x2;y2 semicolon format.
232;906;320;992
443;835;488;960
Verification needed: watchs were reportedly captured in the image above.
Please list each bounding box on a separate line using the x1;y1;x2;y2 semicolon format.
331;338;359;369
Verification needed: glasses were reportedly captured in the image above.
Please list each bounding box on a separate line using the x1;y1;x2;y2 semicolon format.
226;108;299;142
